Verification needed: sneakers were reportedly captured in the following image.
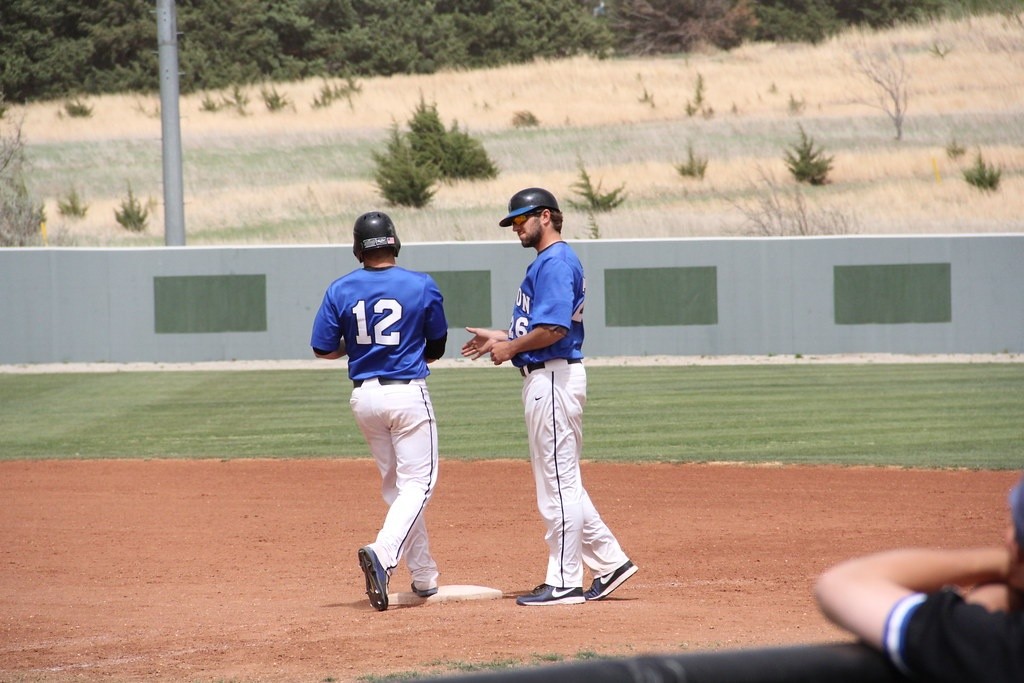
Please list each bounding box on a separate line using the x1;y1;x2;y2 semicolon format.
411;581;438;597
358;547;390;611
516;583;586;605
584;559;639;601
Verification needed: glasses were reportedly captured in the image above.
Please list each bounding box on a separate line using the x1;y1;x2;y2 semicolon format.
513;210;543;227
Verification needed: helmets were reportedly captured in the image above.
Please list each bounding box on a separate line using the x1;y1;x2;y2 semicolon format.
499;187;560;227
353;212;401;263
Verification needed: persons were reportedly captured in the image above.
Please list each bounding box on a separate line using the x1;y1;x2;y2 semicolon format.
816;478;1024;683
461;187;638;606
310;211;448;611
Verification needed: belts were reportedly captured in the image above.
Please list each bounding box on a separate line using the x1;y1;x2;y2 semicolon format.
521;356;582;377
353;375;411;388
